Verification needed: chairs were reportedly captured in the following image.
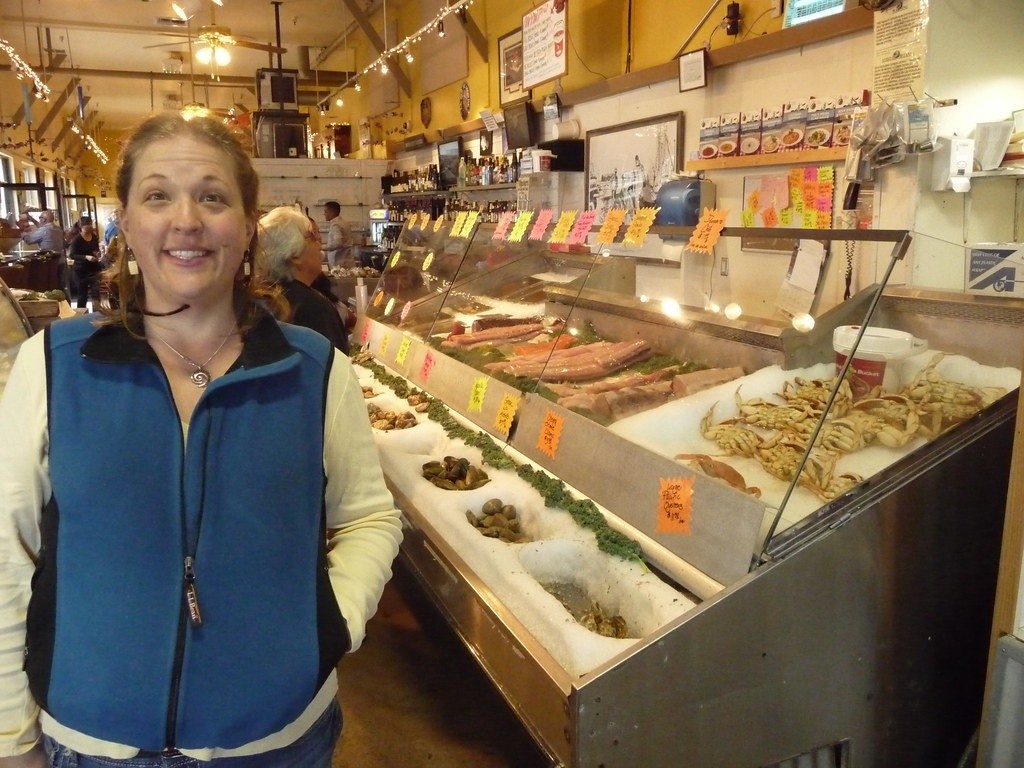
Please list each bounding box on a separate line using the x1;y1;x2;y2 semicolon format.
0;253;60;290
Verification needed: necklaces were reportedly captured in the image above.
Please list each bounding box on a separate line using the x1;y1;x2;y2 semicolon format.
141;318;238;387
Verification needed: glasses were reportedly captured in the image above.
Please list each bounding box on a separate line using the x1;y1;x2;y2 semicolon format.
109;273;190;316
39;216;43;219
305;227;319;241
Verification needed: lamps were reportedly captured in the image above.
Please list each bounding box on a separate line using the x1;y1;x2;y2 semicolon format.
437;20;446;39
173;0;202;21
197;41;237;82
404;42;414;64
381;57;389;75
320;104;325;116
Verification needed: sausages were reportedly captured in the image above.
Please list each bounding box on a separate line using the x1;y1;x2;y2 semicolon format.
442;323;652;382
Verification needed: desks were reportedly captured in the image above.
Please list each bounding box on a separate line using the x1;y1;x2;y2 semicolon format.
0;250;40;264
357;245;392;269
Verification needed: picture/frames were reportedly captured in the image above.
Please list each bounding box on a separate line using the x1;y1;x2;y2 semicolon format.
678;47;708;92
438;136;461;186
584;110;685;224
497;25;532;109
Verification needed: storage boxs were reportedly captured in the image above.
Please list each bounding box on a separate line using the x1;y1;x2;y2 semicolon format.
966;241;1024;297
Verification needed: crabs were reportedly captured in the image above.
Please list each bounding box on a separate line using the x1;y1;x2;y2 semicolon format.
673;348;1008;504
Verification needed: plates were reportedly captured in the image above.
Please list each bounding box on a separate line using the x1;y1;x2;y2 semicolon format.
700;126;853;158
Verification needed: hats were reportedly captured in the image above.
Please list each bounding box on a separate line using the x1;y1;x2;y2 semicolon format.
80;217;92;226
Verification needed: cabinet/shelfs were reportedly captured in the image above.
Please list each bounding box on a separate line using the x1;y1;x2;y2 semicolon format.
378;183;517;250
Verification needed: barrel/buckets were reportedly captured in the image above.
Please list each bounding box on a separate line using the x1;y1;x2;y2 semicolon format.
832;326;929;401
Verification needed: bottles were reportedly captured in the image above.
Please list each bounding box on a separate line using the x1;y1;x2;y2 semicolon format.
387;152;521;221
289;127;298;158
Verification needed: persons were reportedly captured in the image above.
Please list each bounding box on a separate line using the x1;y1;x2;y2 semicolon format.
1;113;402;768
248;198;352;358
22;207;118;313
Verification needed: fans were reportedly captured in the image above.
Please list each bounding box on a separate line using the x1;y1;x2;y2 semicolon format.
143;1;287;56
